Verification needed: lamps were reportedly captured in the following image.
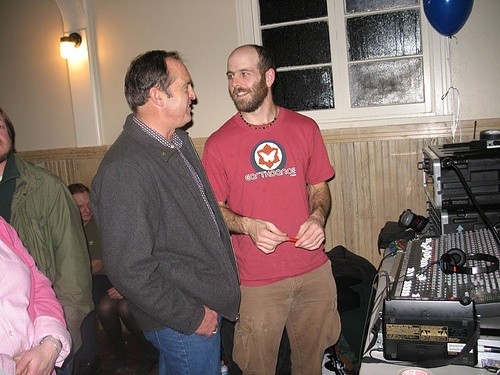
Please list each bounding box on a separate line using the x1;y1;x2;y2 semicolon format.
59;32;82;61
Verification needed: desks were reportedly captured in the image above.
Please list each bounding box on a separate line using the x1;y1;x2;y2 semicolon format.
357;247;500;375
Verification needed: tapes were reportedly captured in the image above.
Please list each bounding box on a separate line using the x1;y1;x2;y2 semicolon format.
479;129;500;140
395;367;433;375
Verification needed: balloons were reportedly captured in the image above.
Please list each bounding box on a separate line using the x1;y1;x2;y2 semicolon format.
423;0;473;38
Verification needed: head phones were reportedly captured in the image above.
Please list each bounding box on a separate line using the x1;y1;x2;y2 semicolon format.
439;248;499;275
398;209;431;233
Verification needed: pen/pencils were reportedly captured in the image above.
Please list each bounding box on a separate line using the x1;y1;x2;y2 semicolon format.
289;237;298;242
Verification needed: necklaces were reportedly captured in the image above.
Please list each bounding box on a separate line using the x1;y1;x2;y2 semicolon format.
240;105;277;130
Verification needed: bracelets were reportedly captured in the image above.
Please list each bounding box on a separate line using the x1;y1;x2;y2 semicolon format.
41;337;62;355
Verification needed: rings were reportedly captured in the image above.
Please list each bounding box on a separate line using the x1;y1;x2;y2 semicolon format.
212;328;218;334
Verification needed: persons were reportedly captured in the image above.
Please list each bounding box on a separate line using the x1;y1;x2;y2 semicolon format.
0;108;96;375
91;50;241;375
0;216;73;375
66;183;345;374
202;44;341;374
